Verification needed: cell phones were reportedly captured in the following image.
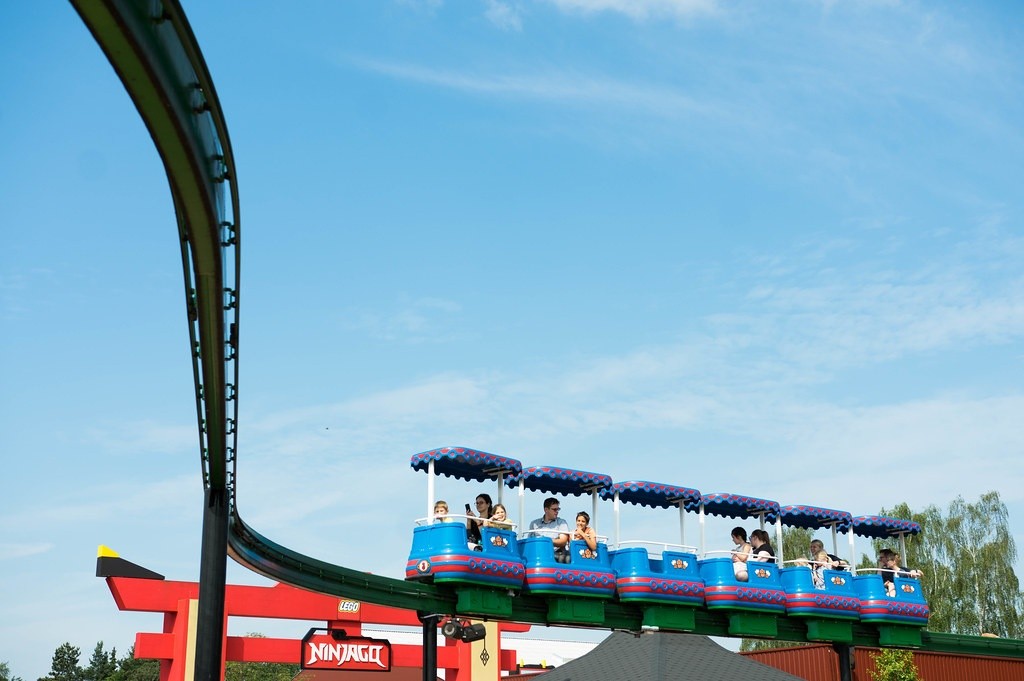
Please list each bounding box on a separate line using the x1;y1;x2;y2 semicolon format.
465;504;470;514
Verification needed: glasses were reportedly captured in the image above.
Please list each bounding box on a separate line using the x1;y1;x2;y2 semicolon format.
548;508;561;511
474;501;486;506
879;557;887;560
749;535;757;541
883;559;893;563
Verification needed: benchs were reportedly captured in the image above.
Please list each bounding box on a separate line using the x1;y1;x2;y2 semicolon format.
407;521;927;606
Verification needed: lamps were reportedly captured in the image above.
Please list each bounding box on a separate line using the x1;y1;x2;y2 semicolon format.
440;614;487;644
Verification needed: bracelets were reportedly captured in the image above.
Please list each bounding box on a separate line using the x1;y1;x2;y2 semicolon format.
838;560;840;564
477;523;483;526
915;569;917;573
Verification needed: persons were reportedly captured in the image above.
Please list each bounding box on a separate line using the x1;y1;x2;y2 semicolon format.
573;510;597;551
875;548;924;597
794;540;849;590
483;503;512;530
527;497;569;553
433;501;452;522
466;492;494;544
730;527;775;582
